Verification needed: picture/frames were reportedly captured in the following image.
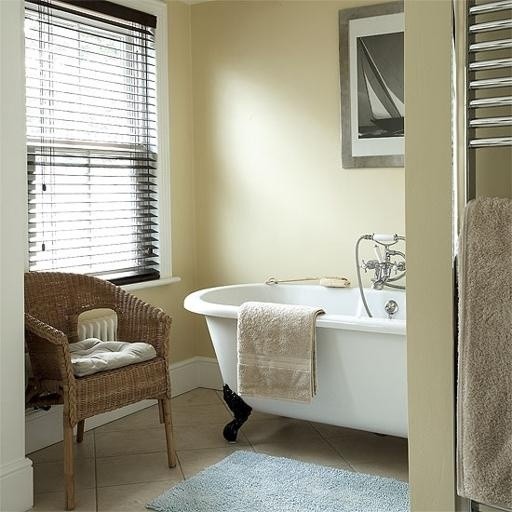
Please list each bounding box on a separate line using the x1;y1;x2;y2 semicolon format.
337;1;406;170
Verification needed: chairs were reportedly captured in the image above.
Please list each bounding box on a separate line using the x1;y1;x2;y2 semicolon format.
25;272;177;511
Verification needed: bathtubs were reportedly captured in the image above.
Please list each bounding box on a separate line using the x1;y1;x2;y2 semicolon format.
184;280;407;442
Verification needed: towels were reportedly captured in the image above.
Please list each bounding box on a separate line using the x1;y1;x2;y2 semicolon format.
459;195;512;511
237;302;326;404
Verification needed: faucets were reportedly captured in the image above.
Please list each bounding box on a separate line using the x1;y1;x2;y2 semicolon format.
361;257;405;290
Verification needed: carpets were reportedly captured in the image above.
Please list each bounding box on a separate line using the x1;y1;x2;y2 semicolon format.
145;448;409;512
